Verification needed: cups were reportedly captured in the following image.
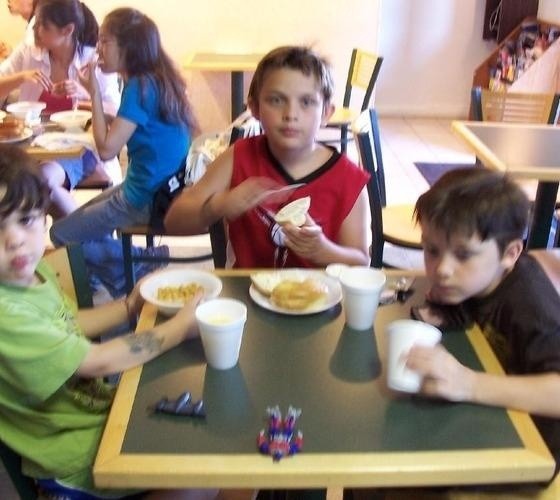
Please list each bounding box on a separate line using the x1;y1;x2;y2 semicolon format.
385;317;444;396
196;297;247;373
340;262;387;331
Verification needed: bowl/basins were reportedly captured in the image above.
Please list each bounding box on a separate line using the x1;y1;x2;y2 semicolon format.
5;100;47;125
138;265;226;321
327;262;349;281
49;109;95;130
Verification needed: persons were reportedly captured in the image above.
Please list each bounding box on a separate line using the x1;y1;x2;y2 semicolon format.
1;0;43;60
162;40;377;274
349;162;559;498
48;7;199;301
0;143;265;499
1;1;127;219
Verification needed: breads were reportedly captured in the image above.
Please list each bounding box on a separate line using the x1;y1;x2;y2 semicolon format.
275;198;311;226
249;267;281;294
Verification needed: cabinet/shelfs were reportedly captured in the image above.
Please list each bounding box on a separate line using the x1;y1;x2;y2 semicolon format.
481;0;539;44
467;18;560;124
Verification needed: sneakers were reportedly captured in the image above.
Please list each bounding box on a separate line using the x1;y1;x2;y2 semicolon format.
134;245;170;284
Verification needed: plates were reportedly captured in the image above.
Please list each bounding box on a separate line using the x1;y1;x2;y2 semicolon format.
249;272;344;318
0;127;33;145
